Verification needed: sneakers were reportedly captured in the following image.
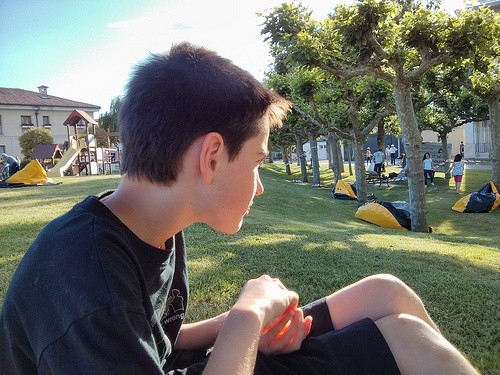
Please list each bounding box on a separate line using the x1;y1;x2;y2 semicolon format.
431;182;434;185
424;185;427;188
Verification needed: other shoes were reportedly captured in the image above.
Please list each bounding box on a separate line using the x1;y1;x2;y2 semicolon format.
456;191;460;194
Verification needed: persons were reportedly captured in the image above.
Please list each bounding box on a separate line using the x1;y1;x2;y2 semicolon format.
0;161;8;181
459;140;465;159
361;143;406;177
422;153;435;187
0;39;485;375
444;152;464;195
109;142;117;162
1;151;20;176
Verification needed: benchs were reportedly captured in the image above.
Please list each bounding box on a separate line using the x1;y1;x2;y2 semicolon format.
365;170;391;188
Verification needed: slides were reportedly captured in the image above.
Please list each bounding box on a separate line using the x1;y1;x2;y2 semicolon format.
46;146;83;177
